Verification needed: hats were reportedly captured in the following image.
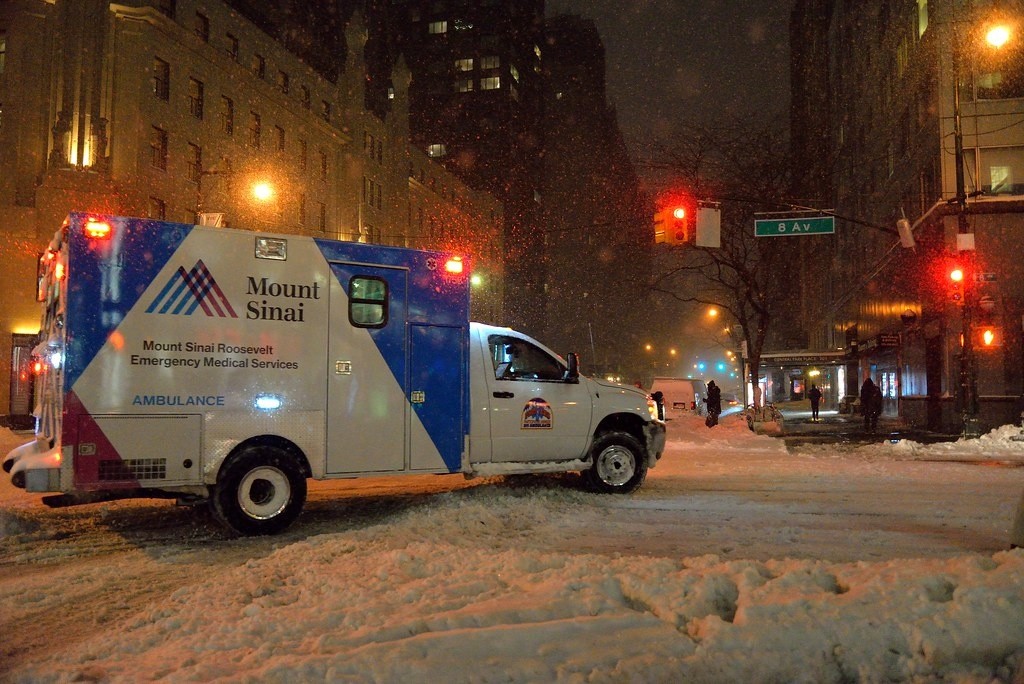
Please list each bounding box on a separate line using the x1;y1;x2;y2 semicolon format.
707;380;715;385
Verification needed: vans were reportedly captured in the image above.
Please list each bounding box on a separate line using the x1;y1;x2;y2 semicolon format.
649;377;709;421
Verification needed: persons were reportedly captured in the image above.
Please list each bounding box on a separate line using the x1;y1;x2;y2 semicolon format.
704;381;721;426
861;378;877;435
809;385;820;419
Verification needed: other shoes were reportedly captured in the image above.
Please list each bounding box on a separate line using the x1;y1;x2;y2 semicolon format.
872;430;876;434
864;430;869;434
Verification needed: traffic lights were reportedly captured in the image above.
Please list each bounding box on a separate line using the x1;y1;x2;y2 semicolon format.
665;204;690;243
977;324;996;347
948;265;965;305
653;206;667;245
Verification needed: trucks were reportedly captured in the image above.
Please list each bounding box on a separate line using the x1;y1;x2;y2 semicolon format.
1;206;668;537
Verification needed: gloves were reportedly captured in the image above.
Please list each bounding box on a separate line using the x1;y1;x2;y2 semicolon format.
702;398;706;402
